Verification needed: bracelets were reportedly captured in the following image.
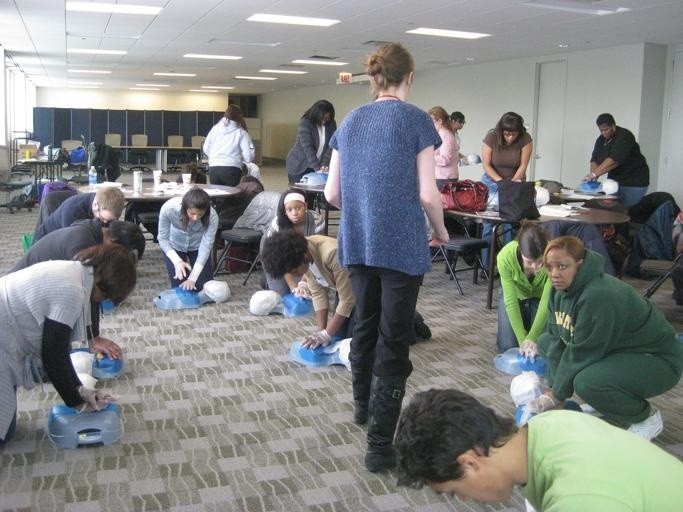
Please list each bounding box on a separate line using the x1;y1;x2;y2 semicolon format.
76;402;89;415
591;172;598;178
541;394;556;407
297;281;309;289
317;328;332;343
290;287;299;294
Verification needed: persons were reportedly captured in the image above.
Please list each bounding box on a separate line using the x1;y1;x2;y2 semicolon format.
155;186;220;292
428;105;458;192
528;235;681;441
317;36;451;475
581;111;651;213
201;103;257;186
445;111;466;167
32;186;127;247
479;111;534;279
1;242;139;448
391;383;682;512
494;219;563;353
233;161;265;189
1;220;146;384
284;99;338;189
261;226;433;351
259;189;317;298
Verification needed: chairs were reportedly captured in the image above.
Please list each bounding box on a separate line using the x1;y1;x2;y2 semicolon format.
62;134;281;286
617;191;683;304
0;181;33;214
419;213;495;295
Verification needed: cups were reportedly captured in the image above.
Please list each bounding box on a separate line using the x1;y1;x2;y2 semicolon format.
152;170;162;189
182;173;191;189
133;171;142;192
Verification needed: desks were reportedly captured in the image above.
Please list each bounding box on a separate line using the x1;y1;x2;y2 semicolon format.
445;190;633;309
22;156;65;203
288;185;341;236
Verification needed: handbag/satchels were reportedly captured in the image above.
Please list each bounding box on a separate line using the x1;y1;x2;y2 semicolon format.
441;179;488;212
497;179;541;220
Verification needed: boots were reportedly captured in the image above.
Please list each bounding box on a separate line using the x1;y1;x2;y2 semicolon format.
351;365;406;473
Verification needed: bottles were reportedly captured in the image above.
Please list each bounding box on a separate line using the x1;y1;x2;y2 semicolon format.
87;165;96;189
46;145;51;162
25;150;30;160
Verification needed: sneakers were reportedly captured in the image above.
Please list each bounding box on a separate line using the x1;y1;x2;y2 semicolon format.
414;312;431;339
581;404;663;442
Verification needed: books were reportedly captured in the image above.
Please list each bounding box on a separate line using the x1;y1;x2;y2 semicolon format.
92;181;133;188
